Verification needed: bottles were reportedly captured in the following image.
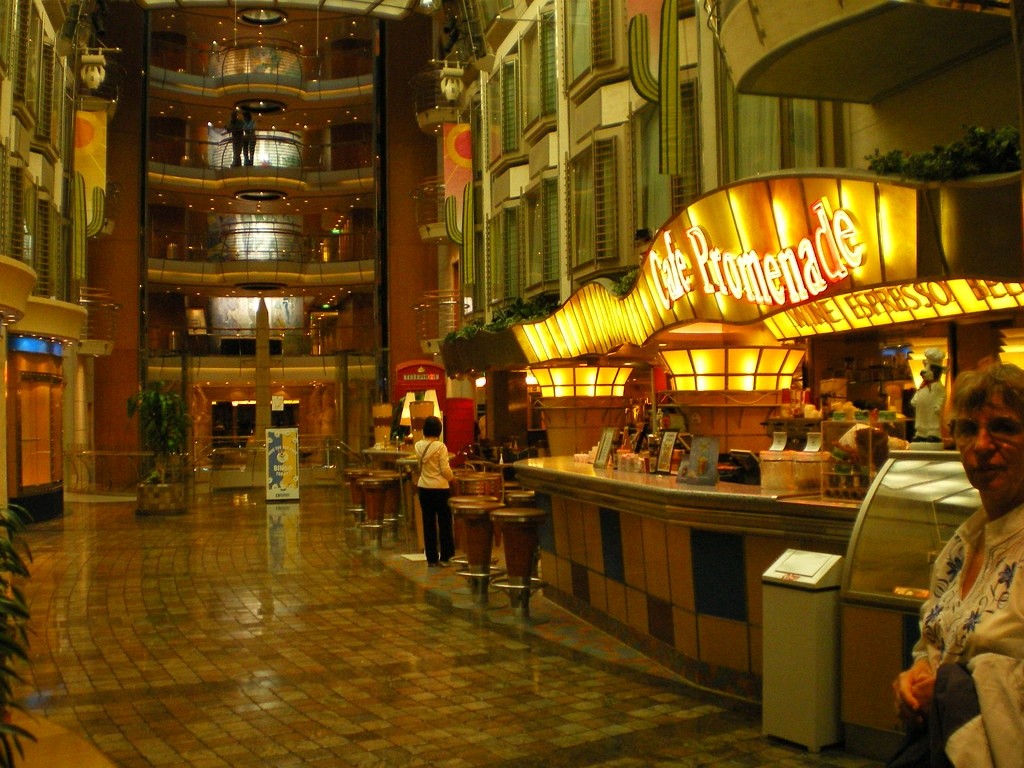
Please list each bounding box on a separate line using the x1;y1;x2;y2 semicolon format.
656;409;663;429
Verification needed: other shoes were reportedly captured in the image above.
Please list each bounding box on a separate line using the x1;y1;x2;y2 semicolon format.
426;559;453;568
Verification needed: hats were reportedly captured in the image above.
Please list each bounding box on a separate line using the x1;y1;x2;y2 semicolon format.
925;347;945;366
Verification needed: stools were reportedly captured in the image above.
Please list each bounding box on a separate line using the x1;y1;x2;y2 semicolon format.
490;507;553;627
450;467;535;558
343;468;407;550
448;495;507;595
451;502;507;610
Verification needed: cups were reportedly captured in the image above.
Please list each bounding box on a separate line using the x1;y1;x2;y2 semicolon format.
697;458;709;475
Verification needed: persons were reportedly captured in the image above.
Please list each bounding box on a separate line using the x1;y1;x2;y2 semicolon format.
241;110;256;167
908;348;946;443
224;110;244;169
413;417;461;567
889;362;1024;768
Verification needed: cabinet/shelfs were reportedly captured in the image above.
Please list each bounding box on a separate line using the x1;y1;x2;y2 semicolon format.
836;457;983;766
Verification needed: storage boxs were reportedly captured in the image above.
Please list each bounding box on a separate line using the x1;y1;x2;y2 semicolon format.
825;398;846;411
759;451;830;498
820;378;847;397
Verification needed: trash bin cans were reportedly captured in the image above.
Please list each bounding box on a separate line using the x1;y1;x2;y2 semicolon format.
756;546;847;752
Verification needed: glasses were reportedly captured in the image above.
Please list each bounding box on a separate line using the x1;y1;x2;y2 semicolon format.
946;416;1024;451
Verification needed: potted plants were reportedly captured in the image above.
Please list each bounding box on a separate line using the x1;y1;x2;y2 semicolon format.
124;379;196;515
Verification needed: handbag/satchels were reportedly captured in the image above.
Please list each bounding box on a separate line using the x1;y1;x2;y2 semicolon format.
411;468;422;484
